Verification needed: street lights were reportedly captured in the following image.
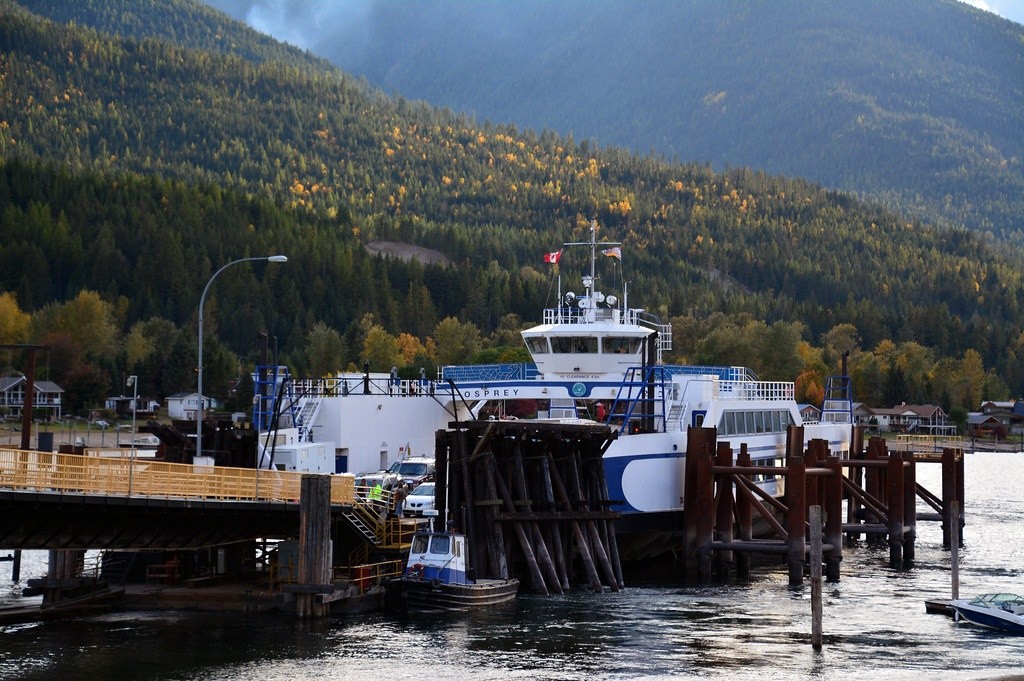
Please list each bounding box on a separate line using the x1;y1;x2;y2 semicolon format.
196;255;289;456
126;375;138;496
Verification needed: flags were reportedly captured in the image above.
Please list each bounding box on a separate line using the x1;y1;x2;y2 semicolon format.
544;248;563;264
602;246;622;261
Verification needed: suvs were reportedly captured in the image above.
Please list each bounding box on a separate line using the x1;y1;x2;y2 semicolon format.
388;454;434;488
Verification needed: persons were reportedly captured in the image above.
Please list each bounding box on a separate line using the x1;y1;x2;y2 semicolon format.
578;340;588;352
356;479;406;545
595;402;605;423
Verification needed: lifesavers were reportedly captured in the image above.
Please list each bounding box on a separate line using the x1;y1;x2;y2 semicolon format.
408;564;424;582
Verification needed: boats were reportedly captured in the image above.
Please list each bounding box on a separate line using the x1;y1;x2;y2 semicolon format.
947;592;1024;634
118;435;159;449
434;220;854;586
375;442;519;615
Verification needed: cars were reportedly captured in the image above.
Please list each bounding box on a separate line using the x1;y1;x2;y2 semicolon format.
402;481;436;518
353;472;402;498
